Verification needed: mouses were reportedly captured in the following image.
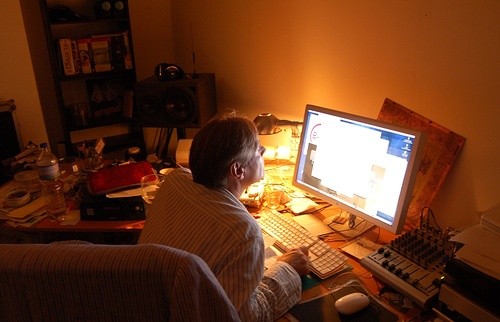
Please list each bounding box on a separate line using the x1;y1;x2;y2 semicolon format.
335;292;369;315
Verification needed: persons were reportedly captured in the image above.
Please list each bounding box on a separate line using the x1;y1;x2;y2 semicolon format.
135;113;311;322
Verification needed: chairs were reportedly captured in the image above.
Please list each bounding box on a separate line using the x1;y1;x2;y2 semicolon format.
0;226;243;322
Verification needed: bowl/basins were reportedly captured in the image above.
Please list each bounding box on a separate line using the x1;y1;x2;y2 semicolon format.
5;189;30;206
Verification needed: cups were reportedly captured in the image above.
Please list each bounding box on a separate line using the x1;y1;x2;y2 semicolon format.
141;174;165;205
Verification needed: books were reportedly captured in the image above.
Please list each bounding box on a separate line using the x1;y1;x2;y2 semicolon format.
56;32;133;76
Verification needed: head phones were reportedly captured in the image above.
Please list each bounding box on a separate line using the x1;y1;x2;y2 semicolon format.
154;63;184;81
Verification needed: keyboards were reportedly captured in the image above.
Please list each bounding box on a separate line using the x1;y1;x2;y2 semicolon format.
256;209;349;280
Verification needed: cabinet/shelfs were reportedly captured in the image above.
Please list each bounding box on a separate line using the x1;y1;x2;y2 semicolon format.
39;0;141;159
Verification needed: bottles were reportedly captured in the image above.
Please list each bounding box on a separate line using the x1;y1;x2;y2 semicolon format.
36;143;66;218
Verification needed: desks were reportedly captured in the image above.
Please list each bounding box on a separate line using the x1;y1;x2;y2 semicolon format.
0;145;500;322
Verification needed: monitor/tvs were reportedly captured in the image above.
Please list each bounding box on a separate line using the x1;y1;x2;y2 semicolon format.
292;104;423;240
